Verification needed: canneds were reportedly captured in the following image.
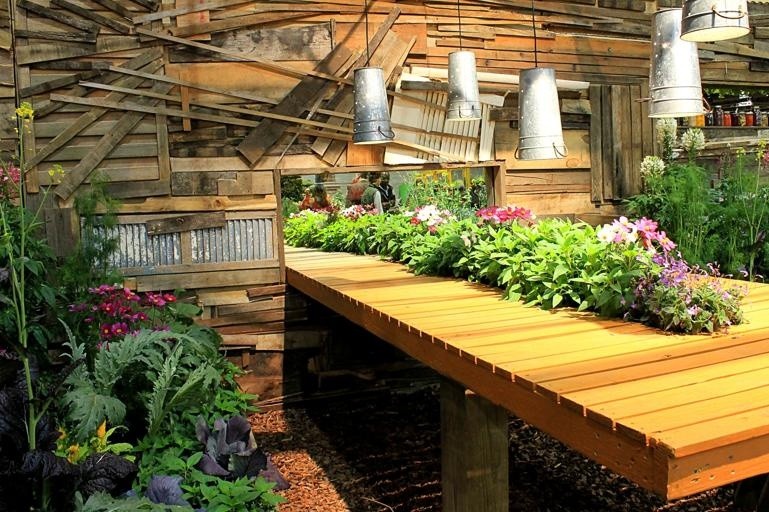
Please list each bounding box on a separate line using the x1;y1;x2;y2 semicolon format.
676;106;769;126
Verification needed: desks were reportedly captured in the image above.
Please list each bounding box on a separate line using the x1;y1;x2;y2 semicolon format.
287;214;769;508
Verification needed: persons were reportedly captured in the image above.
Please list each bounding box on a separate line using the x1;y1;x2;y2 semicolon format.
346;172;369;204
360;172;384;216
377;172;398;214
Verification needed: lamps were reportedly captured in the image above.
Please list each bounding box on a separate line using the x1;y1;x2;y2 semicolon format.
509;0;569;165
344;3;398;149
438;0;486;123
644;0;754;127
